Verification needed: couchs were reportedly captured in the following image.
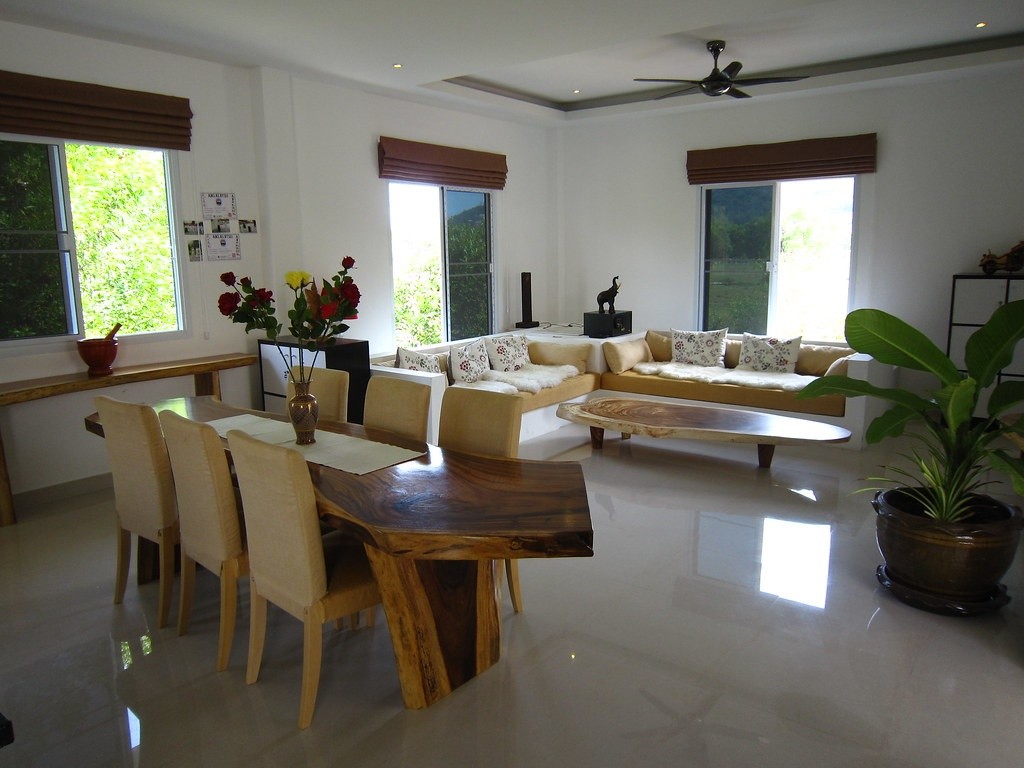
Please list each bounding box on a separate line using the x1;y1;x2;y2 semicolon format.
600;328;896;451
368;334;600;440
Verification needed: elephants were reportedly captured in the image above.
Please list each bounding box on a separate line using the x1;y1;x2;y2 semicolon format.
596;276;622;314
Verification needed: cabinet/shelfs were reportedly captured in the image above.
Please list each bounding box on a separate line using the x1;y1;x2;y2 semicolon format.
941;272;1024;432
258;333;371;426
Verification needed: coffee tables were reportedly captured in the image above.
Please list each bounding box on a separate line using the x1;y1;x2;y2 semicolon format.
556;389;851;467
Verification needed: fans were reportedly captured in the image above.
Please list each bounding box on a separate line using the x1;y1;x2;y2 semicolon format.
634;40;810;99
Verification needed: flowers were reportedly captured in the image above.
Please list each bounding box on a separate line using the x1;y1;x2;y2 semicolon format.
218;256;360;382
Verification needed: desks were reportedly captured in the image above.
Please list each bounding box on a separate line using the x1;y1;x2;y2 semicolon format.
85;393;594;712
0;350;257;526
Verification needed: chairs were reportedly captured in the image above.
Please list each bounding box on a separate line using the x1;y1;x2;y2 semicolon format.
97;367;523;728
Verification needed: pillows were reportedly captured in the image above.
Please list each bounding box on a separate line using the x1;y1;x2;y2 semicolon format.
602;338;654;375
735;332;802;374
528;338;592;376
671;327;729;369
395;347;441;373
483;334;531;372
447;337;490;385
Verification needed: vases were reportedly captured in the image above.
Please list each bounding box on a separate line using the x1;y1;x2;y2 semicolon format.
289;380;320;446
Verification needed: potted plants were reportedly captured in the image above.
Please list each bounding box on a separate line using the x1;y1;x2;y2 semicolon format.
800;297;1024;616
